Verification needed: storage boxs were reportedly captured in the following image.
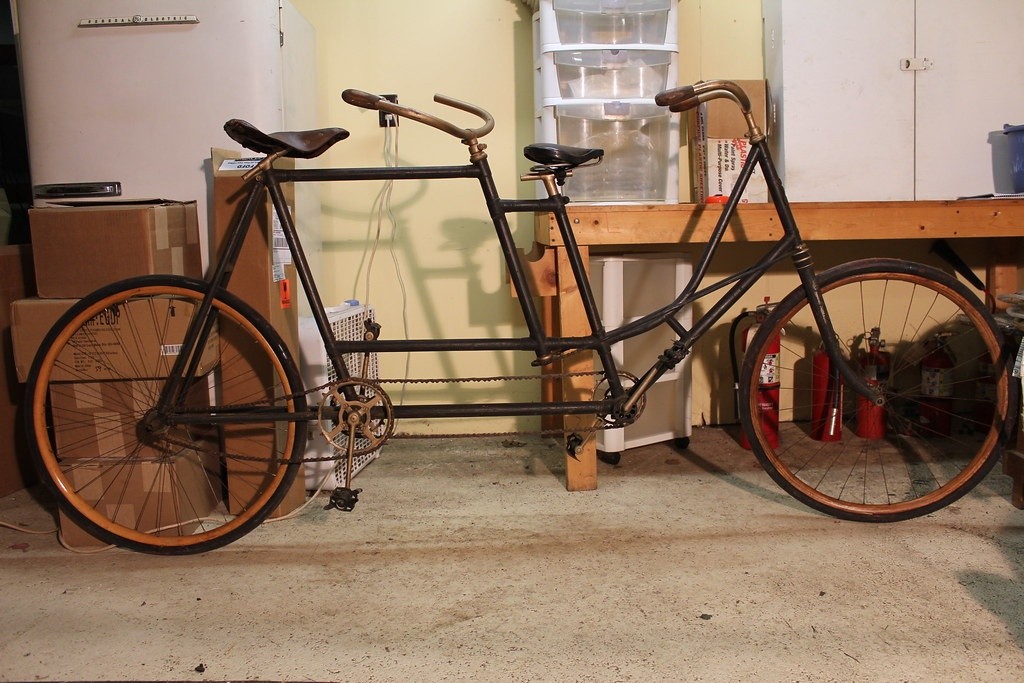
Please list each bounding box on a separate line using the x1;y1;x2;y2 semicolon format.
9;303;222;383
691;76;769;201
28;200;203;298
536;2;679;203
57;432;221;548
49;375;211;459
205;148;308;516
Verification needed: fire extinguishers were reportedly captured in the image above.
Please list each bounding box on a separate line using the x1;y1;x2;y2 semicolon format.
919;328;961;435
856;327;889;438
730;294;780;451
974;352;997;432
810;323;846;442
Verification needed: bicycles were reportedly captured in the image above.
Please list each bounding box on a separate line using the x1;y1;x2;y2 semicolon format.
25;77;1021;556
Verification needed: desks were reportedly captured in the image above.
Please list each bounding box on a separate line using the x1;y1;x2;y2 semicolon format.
512;194;1023;490
